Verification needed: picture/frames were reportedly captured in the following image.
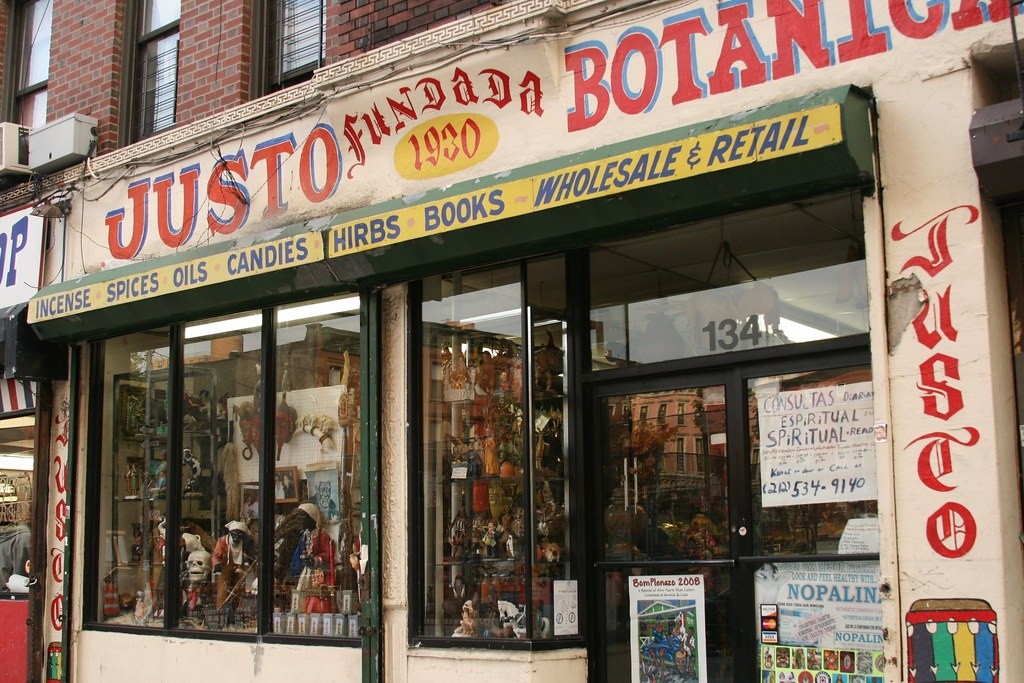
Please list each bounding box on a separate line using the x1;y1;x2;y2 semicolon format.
274;466;300;503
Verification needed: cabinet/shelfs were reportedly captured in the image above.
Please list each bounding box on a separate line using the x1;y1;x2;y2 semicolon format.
436;305;568;639
113;363;218;610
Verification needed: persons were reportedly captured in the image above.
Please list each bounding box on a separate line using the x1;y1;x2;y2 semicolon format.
440;341;500;638
213;521;254;622
125;389;210;496
0;524;31;594
291;502;337;614
274;474;293;498
309;473;337;521
186;550;209;582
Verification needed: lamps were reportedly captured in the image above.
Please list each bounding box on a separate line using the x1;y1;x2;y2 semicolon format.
30;204;64;219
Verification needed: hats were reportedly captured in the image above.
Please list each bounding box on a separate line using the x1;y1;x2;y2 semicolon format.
225;521;247;534
298;503;322;528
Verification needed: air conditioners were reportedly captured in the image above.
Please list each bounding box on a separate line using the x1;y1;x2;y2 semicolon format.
0;121;32;176
